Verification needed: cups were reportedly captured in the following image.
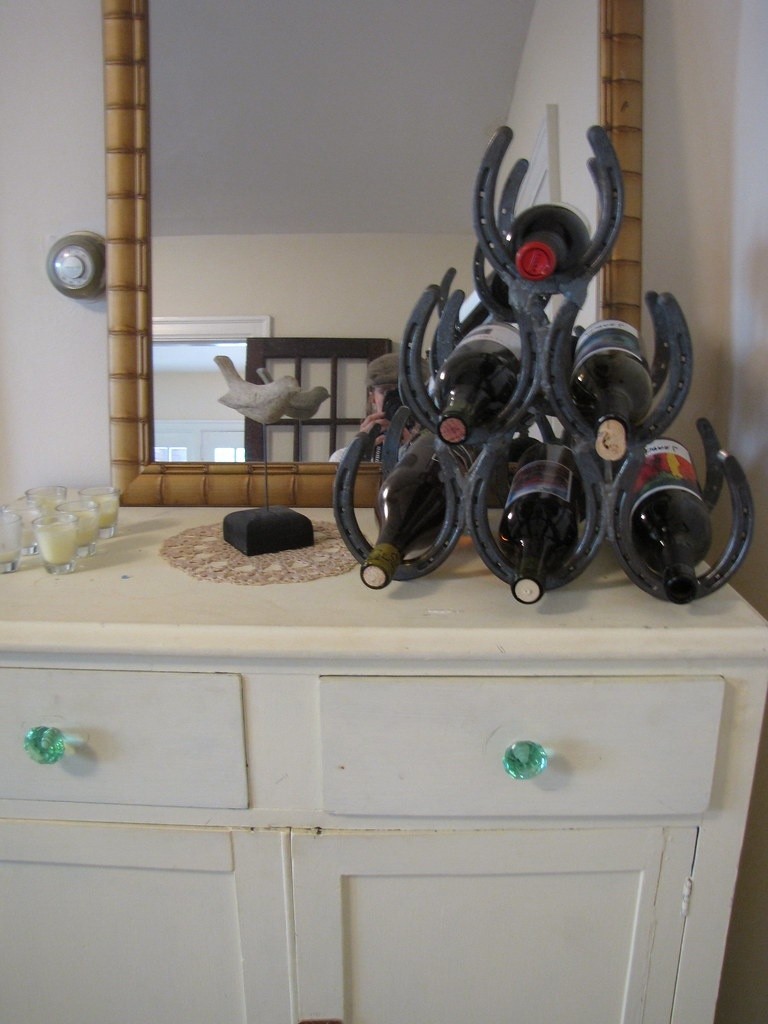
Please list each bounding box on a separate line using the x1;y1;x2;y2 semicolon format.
0;495;44;556
24;486;68;514
32;511;81;576
55;499;98;559
78;484;119;539
0;512;26;574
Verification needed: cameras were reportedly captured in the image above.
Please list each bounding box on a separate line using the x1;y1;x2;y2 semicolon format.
382;390;416;430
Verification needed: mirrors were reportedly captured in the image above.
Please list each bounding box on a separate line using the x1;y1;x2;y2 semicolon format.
104;0;646;505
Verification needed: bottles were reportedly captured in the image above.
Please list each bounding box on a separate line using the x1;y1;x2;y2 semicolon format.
508;202;592;282
358;431;470;589
432;313;529;445
571;320;654;460
628;436;714;604
498;442;585;604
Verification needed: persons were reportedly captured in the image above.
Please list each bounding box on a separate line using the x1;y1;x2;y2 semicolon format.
328;352;431;464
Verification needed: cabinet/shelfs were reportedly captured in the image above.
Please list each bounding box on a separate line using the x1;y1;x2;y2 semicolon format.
0;494;768;1024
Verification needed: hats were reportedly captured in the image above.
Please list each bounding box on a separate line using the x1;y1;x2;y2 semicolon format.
367;353;430;385
